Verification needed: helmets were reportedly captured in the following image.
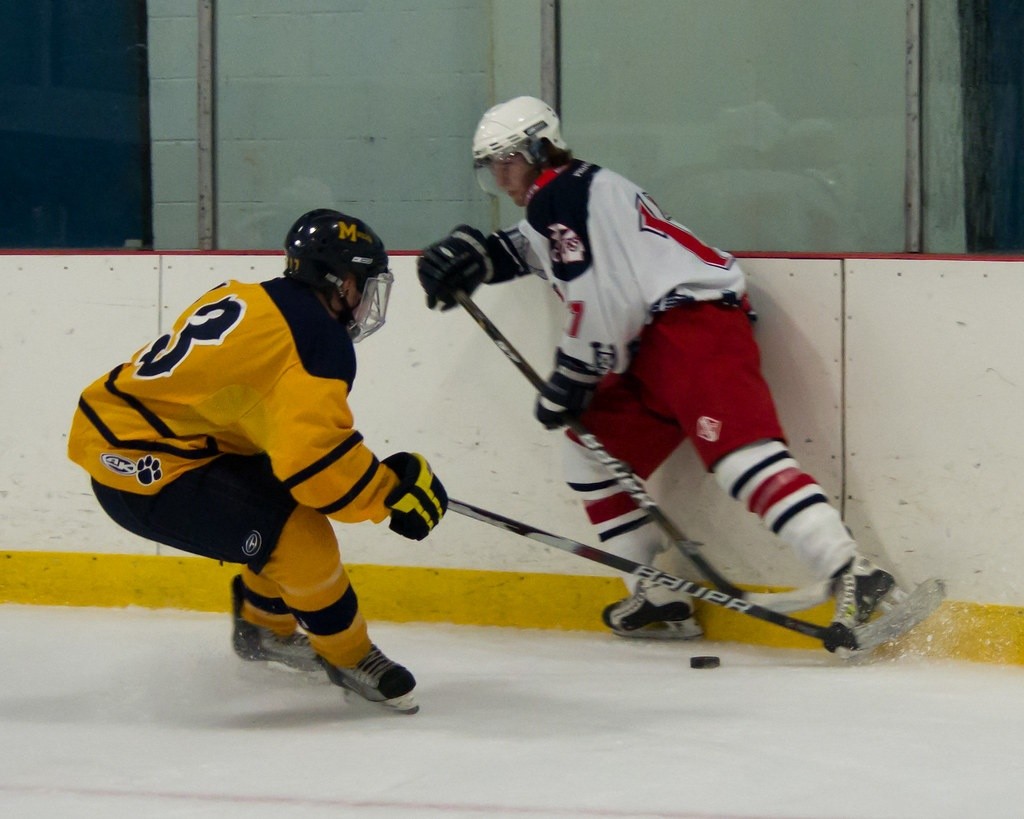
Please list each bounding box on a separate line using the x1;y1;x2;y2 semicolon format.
471;95;572;169
284;208;388;292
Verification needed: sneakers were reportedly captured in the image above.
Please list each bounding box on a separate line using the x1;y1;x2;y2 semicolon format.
231;619;326;672
821;554;895;654
600;578;697;632
315;642;418;702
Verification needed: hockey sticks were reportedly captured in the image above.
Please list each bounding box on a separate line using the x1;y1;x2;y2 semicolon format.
452;293;839;610
440;495;945;651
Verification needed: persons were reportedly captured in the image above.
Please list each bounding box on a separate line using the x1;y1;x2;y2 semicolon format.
69;209;448;699
415;93;895;653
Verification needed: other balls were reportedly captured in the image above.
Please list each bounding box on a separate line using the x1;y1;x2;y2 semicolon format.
688;656;721;670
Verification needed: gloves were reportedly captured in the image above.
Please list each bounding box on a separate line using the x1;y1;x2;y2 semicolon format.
417;222;496;312
533;344;603;430
